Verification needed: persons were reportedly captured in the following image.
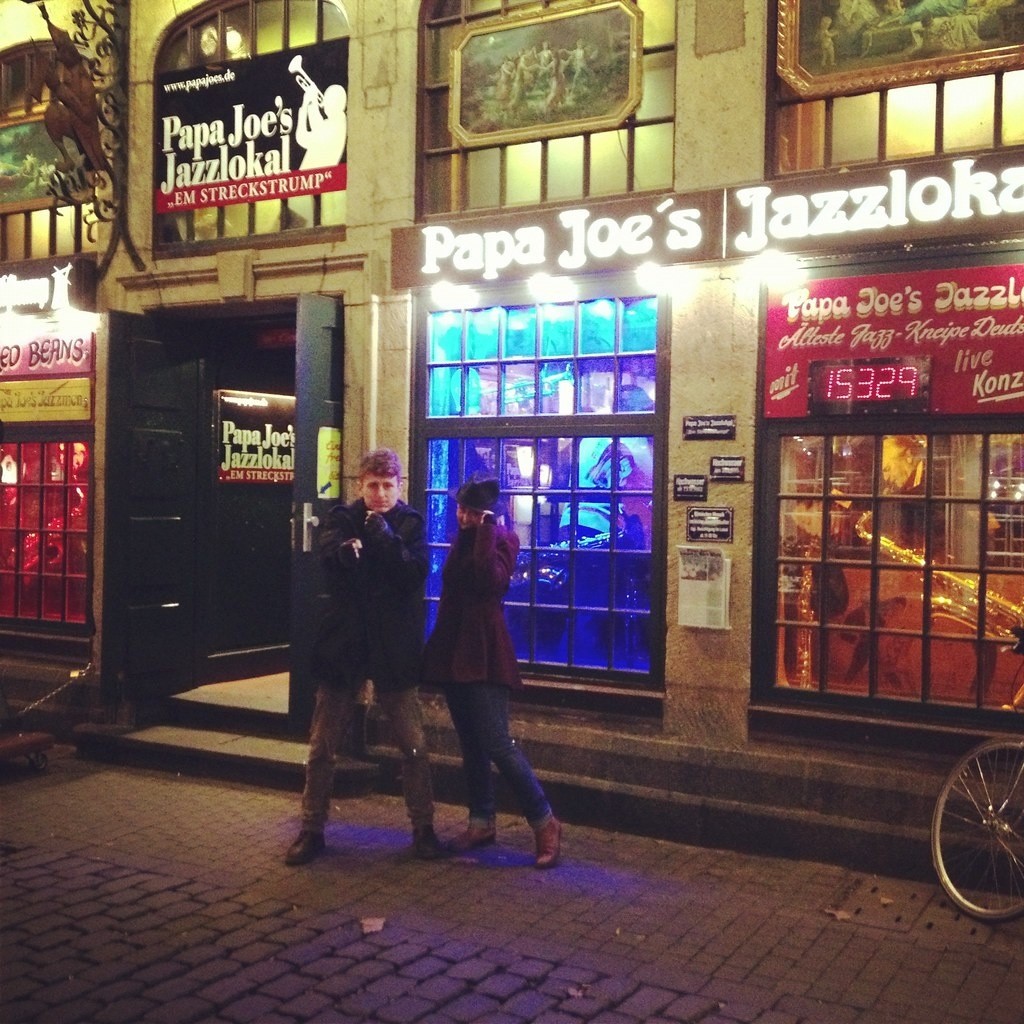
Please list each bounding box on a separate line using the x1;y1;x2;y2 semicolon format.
882;434;927;495
422;476;562;869
813;0;1020;72
283;447;444;866
495;37;602;117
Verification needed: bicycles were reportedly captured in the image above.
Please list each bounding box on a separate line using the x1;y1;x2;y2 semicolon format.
931;625;1024;926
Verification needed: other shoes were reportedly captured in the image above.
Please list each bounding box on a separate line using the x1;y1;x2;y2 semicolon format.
442;826;496;851
413;821;437;857
534;816;562;866
286;830;326;862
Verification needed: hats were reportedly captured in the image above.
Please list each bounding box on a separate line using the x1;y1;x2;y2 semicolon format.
447;470;507;516
